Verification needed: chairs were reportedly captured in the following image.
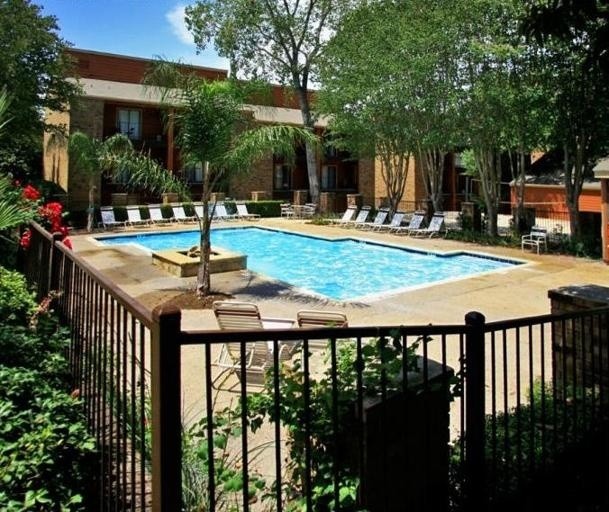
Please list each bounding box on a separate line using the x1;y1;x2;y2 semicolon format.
521;226;548;256
279;202;317;221
210;300;294;393
97;201;261;233
276;310;349;376
323;204;447;239
